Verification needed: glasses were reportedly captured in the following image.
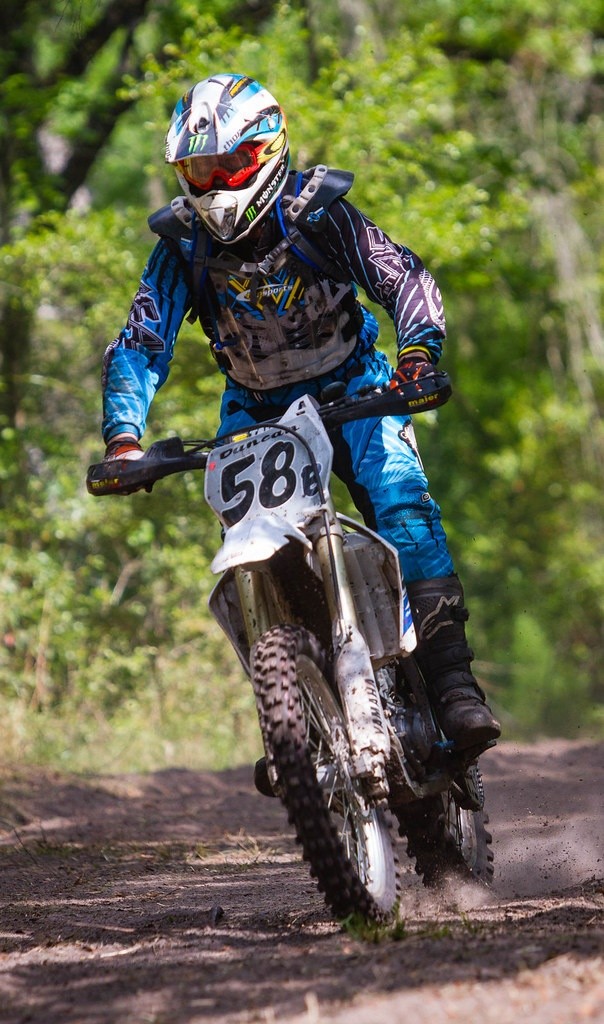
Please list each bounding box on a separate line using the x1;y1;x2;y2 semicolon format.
176;143;260;190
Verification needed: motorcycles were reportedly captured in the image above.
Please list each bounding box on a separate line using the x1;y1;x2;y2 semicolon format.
81;374;498;937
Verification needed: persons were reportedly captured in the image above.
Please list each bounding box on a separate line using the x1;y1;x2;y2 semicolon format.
101;75;505;744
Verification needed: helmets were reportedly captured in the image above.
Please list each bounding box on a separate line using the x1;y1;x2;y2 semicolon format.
165;74;290;246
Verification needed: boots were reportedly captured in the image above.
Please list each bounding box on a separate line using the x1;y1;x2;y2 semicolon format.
404;574;501;745
254;754;279;798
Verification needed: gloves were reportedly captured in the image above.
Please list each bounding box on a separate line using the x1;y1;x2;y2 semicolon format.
101;438;153;497
388;357;442;394
